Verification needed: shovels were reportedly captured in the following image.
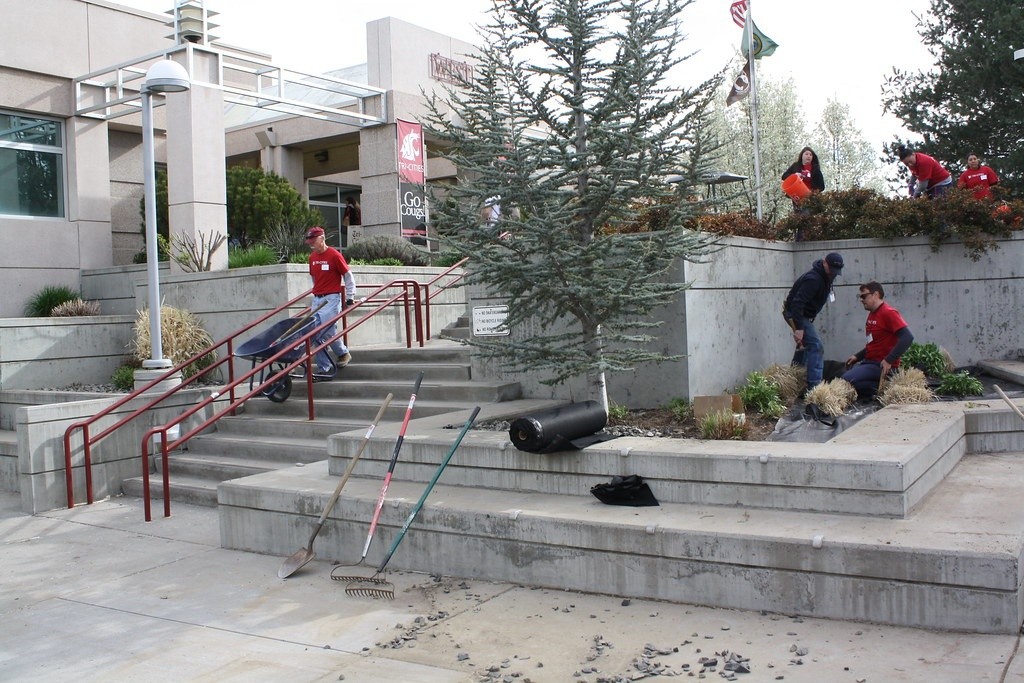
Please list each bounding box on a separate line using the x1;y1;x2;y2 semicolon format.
276;393;394;579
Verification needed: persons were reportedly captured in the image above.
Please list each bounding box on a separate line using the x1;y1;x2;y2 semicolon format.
898;146;953;200
783;252;845;398
840;281;915;401
304;227;356;375
343;197;361;225
957;153;1022;231
782;147;825;214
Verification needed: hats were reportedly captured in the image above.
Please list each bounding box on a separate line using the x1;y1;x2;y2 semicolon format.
305;227;325;244
825;253;843;275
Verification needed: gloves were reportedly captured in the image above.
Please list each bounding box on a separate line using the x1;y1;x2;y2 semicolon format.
346;299;354;305
909;186;914;197
913;193;921;199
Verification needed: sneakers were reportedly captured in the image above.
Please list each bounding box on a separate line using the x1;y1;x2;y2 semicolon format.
336;351;352;368
311;370;333;383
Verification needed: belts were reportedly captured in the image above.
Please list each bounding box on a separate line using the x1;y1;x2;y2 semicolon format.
314;292;339;298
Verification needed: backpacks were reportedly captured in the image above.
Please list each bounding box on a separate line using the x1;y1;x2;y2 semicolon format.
348;205;361;225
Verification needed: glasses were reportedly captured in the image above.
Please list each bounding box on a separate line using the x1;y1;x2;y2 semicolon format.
860;292;874;300
305;235;320;239
828;259;844;269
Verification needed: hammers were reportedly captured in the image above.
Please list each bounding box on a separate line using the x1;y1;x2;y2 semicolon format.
872;367;888;401
786;317;807;352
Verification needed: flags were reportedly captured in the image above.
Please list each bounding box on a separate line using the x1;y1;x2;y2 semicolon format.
725;0;778;108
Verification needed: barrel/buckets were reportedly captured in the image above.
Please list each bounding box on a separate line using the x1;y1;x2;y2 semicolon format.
781;173;812;205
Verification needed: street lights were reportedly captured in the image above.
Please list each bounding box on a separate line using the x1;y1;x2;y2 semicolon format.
139;59;192;370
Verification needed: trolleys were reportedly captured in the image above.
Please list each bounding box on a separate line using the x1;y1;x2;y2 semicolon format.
231;302;349;403
